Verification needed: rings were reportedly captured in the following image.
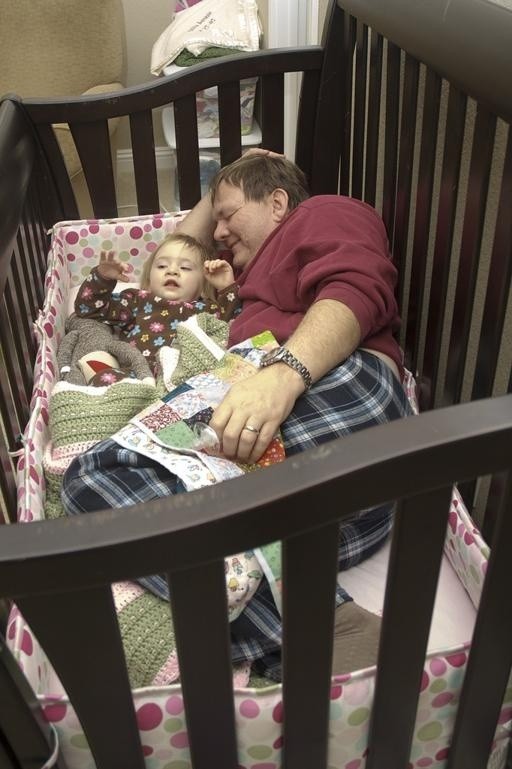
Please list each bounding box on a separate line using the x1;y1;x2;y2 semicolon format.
243;426;260;435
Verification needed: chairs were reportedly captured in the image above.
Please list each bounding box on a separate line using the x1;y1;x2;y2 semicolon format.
0;0;124;220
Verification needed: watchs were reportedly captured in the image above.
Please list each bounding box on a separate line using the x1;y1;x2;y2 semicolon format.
259;346;314;391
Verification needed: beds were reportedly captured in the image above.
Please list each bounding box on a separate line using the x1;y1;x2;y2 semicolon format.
0;1;511;769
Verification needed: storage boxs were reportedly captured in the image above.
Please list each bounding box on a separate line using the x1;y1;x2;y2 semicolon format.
153;24;270;144
161;98;262;210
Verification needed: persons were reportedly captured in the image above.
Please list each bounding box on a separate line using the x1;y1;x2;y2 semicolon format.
59;149;413;680
74;234;242;387
